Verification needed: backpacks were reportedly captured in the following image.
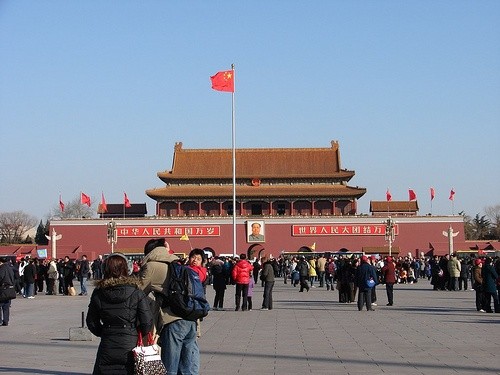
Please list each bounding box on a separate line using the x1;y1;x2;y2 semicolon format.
149;258;210;320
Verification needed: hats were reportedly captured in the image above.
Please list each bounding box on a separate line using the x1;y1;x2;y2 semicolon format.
386;256;392;262
475;258;485;266
361;256;367;262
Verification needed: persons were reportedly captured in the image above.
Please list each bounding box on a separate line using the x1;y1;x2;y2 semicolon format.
0;238;500;375
249;222;264;241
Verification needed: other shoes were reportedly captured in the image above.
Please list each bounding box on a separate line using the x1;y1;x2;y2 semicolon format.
312;285;316;288
260;307;268;311
367;308;375;311
78;293;83;295
0;323;8;326
479;309;486;313
27;296;35;299
212;306;217;310
299;290;303;292
371;303;377;306
217;308;225;311
307;287;309;292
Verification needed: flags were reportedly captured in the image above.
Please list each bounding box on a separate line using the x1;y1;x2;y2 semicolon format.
431;187;435;199
449;189;455;200
386;193;391;201
124;194;131;207
102;195;107;212
81;194;90;207
60;201;64;212
211;70;235;92
409;190;415;200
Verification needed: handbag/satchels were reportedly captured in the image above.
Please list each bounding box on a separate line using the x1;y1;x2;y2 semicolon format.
1;286;16;301
67;286;75;296
52;271;59;280
438;269;444;277
132;331;168;375
